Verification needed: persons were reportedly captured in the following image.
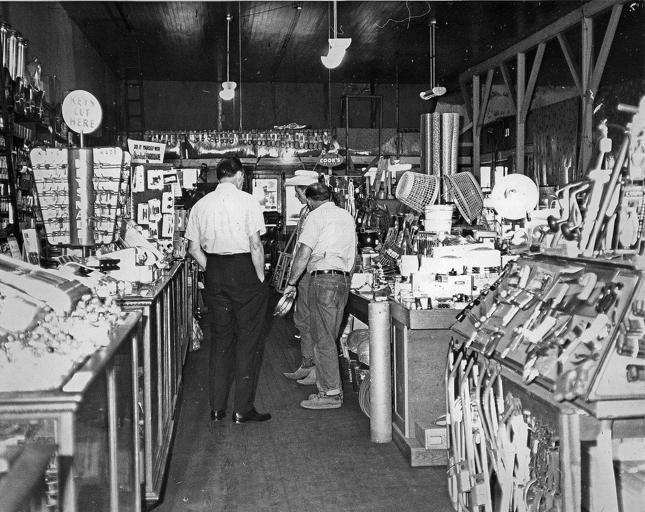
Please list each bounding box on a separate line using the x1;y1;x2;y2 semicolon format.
179;155;271;427
281;182;358;412
282;168;321;386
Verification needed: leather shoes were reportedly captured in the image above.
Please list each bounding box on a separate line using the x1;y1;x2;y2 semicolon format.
233;408;273;424
210;409;227;422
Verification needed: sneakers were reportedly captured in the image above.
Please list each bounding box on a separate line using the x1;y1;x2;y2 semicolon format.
299;387;345;410
282;365;317;385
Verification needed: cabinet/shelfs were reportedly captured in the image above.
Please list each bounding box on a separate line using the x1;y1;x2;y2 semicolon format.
348;290;466;467
2;264;199;512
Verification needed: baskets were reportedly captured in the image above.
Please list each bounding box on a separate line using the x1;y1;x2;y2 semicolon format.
444;171;484;225
394;171;440;214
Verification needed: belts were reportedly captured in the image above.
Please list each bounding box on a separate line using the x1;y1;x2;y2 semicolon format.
311;269;350;277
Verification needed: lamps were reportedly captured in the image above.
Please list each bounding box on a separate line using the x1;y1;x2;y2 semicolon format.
320;0;352;69
418;18;446;100
218;11;237;101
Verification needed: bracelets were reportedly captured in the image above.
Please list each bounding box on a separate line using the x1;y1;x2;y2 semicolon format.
286;283;296;288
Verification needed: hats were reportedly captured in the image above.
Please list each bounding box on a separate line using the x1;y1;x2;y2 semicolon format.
285;170;319;186
